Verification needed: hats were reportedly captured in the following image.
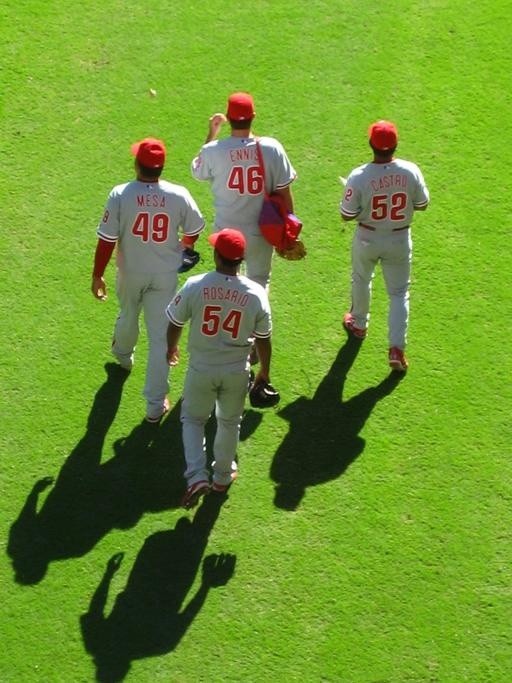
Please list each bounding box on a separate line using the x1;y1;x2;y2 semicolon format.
370;121;398;151
131;137;165;168
226;92;254;121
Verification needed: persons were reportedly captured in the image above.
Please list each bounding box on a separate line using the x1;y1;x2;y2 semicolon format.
90;134;208;423
162;221;274;509
336;118;430;373
193;89;306;364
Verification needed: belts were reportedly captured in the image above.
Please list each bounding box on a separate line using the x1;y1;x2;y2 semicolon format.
359;223;409;231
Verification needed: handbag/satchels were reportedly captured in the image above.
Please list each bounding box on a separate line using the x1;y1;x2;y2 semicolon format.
259;193;302;251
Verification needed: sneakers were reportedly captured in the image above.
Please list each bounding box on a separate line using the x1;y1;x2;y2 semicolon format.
344;312;367;339
180;481;210;511
212;461;237;492
389;347;409;372
145;398;169;423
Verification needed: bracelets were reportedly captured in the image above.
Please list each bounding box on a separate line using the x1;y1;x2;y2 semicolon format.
167;346;178;354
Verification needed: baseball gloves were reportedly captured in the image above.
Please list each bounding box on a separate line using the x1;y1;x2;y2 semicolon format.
248;381;281;407
278;241;306;260
178;249;199;273
